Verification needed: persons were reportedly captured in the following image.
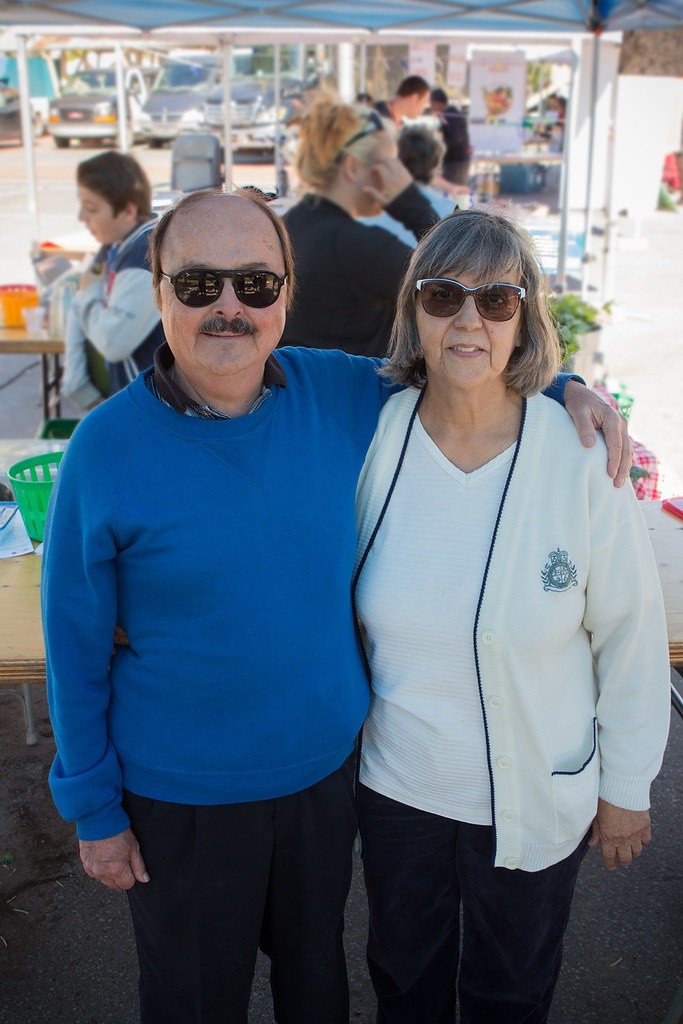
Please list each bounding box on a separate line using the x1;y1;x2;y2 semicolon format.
354;94;391;118
351;208;672;1024
373;74;432;124
383;114;465;238
429;89;471;178
534;93;568;146
33;151;172;408
35;188;633;1024
261;100;445;360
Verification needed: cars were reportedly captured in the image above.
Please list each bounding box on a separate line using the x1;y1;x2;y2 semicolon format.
0;82;43;149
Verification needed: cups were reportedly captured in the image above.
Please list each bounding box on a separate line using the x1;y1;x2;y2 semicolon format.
21;306;45;334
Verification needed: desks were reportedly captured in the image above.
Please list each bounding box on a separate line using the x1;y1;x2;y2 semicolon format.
471;150;565;204
1;538;48;747
1;324;68;421
637;494;683;724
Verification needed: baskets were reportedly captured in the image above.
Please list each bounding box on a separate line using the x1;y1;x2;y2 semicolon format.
8;443;66;549
1;283;40;328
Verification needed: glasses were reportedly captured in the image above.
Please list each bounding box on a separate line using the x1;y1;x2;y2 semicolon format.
413;273;530;322
328;108;387;176
158;265;292;307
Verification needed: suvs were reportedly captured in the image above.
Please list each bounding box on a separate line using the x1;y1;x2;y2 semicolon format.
45;68;140;148
204;71;322;161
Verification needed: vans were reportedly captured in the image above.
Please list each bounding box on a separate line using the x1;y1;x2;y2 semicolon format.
138;46;287;149
